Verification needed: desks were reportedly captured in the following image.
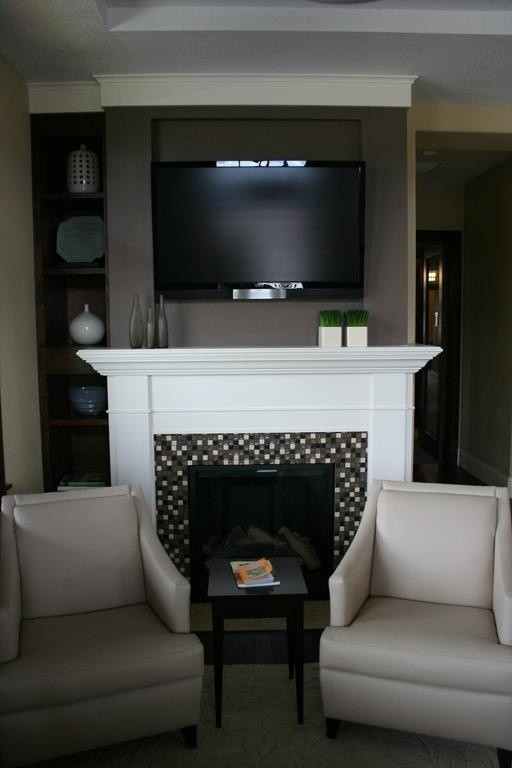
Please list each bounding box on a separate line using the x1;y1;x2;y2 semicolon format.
206;556;311;729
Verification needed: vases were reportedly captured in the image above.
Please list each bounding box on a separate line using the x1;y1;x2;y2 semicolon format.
68;301;106;347
158;294;168;349
68;376;107;421
147;307;156;348
63;142;101;194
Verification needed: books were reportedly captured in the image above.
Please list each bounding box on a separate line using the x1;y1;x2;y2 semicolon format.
60;470;110;486
230;559;280;589
236;557;275;585
57;485;107;492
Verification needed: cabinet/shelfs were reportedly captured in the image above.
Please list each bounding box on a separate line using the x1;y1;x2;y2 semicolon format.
32;113;112;493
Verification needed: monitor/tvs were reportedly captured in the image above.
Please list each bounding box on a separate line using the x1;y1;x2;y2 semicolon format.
148;158;366;304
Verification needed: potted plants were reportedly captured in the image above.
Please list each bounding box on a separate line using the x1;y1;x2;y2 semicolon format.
344;309;368;346
317;309;342;347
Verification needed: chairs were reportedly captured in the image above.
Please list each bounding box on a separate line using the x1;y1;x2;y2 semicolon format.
2;484;205;762
320;480;511;761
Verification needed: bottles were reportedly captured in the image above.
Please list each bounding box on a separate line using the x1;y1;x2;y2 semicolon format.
155;294;168;348
144;307;156;349
